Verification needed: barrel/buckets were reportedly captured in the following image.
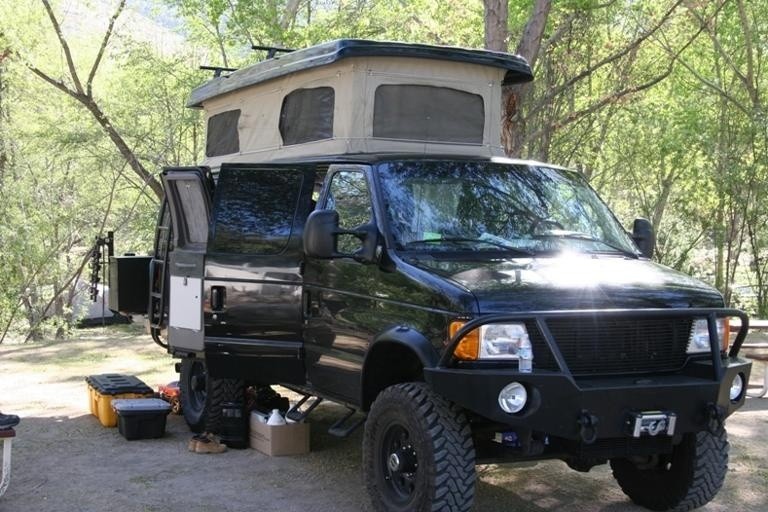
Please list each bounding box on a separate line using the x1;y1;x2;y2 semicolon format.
285;404;301;423
268;408;285;426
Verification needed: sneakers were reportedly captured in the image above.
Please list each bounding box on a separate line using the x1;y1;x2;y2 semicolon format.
188;432;227;454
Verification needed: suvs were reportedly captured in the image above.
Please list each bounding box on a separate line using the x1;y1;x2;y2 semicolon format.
147;39;753;512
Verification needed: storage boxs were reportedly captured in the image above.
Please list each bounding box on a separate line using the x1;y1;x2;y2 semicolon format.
84;374;173;443
249;407;312;458
